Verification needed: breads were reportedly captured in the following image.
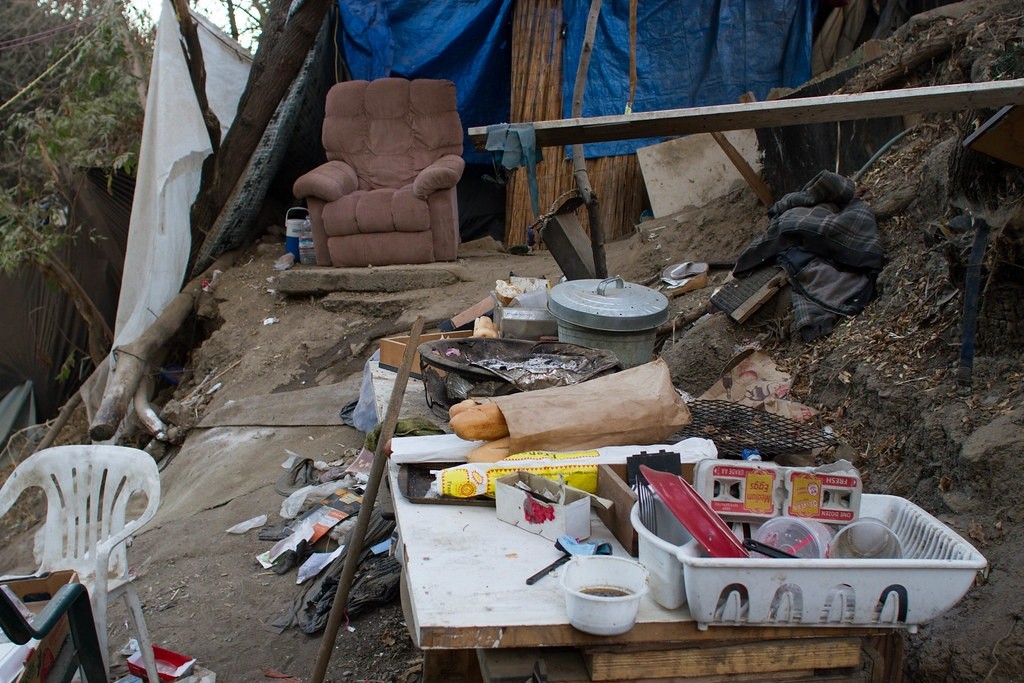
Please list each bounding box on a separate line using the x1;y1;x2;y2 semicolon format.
469;315;497;338
448;399;512;463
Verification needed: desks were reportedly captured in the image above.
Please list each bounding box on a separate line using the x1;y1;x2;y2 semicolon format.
368;358;907;683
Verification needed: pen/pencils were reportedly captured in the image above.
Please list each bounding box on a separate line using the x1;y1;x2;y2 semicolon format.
526;554;571;584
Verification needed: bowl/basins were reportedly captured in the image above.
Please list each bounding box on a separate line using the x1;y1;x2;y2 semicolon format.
559;555;648;635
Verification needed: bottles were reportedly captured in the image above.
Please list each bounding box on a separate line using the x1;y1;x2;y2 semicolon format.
299;214;317;265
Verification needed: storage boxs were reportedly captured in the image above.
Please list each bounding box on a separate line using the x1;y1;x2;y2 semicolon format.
494;470;614;544
630;492;989;625
0;569;79;683
126;643;197;683
596;464;698;557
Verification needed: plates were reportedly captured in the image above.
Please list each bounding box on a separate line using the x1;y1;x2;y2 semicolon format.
639;464;749;558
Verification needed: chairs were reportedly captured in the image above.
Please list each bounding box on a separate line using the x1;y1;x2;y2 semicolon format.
0;444;160;683
0;582;108;683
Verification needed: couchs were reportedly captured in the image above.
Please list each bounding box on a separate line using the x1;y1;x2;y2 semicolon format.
292;78;463;265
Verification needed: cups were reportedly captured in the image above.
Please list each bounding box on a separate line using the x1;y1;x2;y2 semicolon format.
827;516;903;559
750;514;837;557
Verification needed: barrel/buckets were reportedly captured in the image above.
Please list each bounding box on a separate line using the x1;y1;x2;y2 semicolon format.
284;206;310;263
546;276;669;368
